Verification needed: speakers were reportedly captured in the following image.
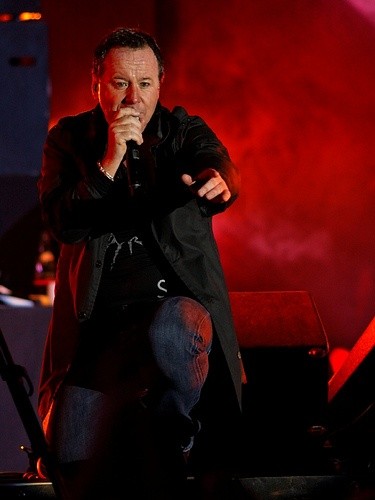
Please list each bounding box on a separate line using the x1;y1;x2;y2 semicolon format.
228;290;331;406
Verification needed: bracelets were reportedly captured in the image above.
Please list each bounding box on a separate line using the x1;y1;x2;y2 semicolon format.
96;159;115;183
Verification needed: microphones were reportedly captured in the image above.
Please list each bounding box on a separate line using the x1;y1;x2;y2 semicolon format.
123;115;143;198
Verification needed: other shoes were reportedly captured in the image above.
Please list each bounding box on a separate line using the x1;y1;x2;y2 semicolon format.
165;434;202;480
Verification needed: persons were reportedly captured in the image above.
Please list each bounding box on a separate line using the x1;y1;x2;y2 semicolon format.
33;27;249;480
